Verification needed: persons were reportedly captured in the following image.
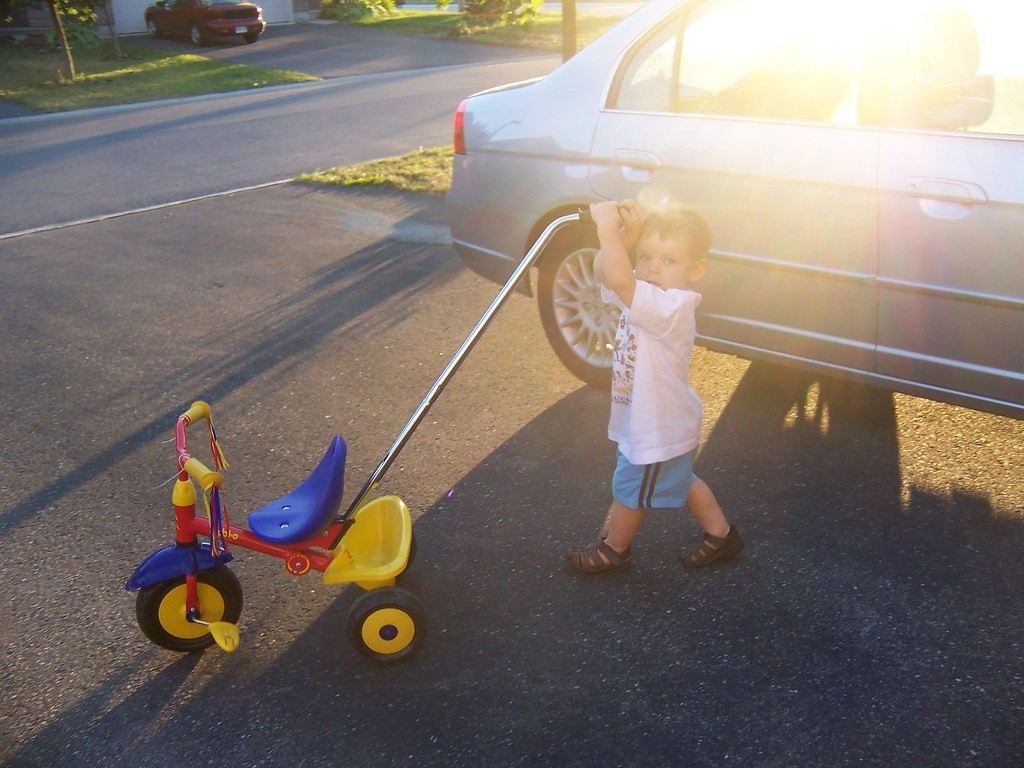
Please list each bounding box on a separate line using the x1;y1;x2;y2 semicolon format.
568;199;743;572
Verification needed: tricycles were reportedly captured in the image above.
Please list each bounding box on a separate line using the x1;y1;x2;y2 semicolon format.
124;400;430;662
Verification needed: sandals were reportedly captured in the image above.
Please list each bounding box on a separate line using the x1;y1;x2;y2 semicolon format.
568;538;631;573
678;526;744;567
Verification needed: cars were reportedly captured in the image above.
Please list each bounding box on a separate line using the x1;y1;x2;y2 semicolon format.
444;0;1024;422
143;0;267;47
